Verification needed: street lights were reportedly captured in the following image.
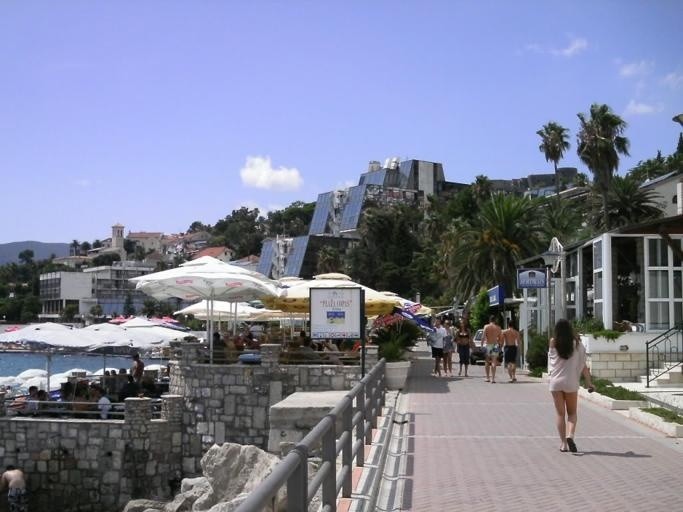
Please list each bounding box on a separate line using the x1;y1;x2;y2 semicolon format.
451;294;459;327
536;248;561;342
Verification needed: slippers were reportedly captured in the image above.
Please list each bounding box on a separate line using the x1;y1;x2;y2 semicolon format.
509;377;516;383
485;378;496;383
559;437;577;452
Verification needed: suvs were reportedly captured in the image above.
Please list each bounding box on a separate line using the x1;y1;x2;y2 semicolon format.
468;328;505;366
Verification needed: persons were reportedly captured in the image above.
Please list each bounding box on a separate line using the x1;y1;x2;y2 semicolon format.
0;464;29;512
19;375;153;418
499;320;521;382
546;318;593;452
480;315;504;383
426;317;476;377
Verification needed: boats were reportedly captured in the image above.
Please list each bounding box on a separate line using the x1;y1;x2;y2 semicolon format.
0;342;72;356
148;345;181;361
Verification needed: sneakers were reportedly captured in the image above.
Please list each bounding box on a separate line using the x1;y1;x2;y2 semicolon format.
431;372;469;377
